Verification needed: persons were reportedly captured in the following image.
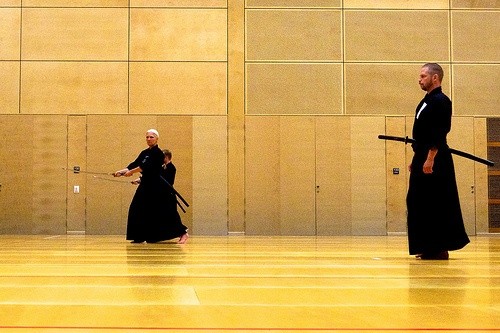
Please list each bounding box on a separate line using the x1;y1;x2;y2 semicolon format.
116;128;189;244
406;62;471;260
